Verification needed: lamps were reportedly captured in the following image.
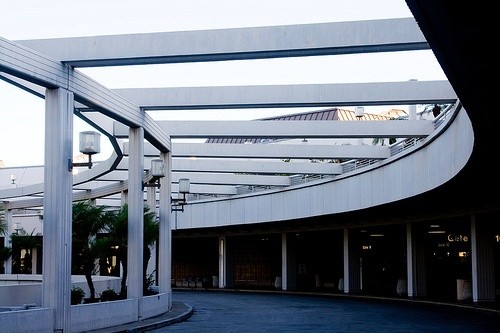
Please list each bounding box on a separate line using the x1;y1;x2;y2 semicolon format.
170;179;190;203
142;159;166;190
68;131;100;172
171;193;188;212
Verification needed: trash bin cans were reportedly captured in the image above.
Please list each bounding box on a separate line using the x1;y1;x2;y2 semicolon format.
274;276;282;288
456;279;470;300
338;278;344;291
212;276;219;287
396;279;406;295
